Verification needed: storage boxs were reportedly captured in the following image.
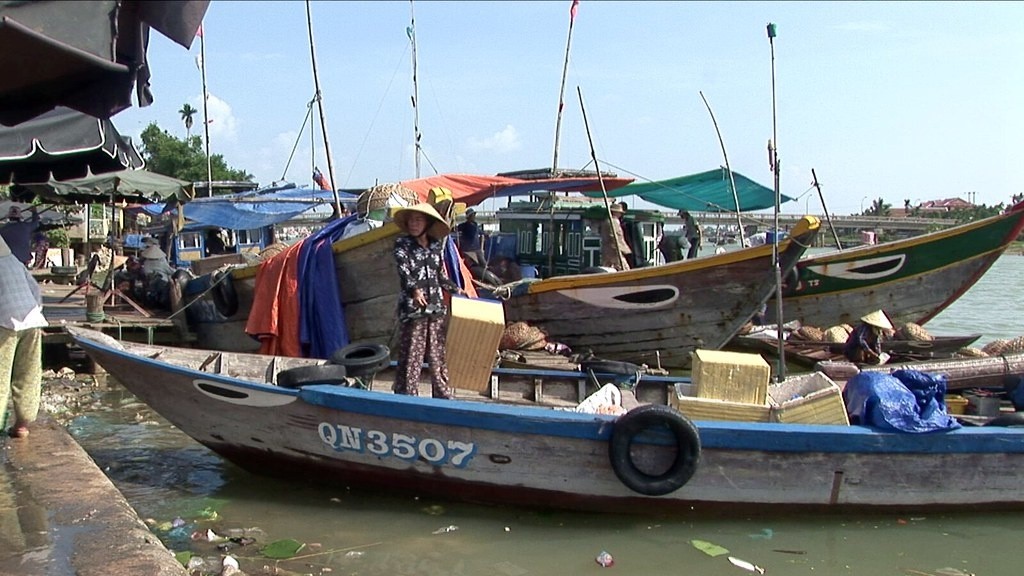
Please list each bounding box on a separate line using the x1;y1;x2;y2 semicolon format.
944;394;968;416
670;381;770;421
445;293;506;396
769;370;849;428
969;396;999;416
689;347;770;404
1000;400;1016;415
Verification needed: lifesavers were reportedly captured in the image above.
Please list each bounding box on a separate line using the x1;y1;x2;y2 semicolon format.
211;272;239;317
277;364;346;386
607;405;702;496
331;341;391;376
470;266;503;285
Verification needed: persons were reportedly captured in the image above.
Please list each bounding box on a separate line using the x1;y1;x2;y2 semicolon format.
451;208;489;269
0;234;43;439
0;201;40;269
599;202;646;270
844;310;894;365
394;202;466;400
678;207;702;258
141;244;177;284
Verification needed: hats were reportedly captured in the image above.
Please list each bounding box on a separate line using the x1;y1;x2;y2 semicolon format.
6;206;23;219
0;235;11;256
677;208;688;215
466;208;476;219
394;203;450;239
610;204;625;213
141;245;166;259
861;310;893;329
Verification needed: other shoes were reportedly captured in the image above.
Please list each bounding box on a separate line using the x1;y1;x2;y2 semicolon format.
8;425;29;438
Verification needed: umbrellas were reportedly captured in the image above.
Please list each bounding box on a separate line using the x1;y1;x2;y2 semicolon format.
0;1;214;305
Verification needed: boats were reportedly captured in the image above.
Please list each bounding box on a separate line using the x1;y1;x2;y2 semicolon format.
755;200;1024;341
59;318;1023;523
465;168;702;282
470;216;817;370
183;221;402;362
